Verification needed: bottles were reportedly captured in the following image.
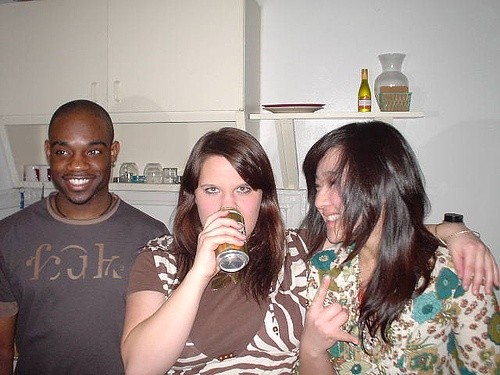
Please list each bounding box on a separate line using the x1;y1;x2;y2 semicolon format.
358;69;371;112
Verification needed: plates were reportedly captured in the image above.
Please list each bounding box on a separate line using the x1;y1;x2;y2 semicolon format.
261;104;326;113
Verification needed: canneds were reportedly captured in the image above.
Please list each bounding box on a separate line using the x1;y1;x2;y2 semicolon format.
214;207;249;272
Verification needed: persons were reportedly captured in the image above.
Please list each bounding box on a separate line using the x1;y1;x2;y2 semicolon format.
0;100;178;375
294;120;500;375
120;127;500;375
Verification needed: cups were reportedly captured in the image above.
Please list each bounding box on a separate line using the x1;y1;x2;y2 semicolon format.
24;165;51;182
118;163;138;184
143;163;163;183
163;168;177;184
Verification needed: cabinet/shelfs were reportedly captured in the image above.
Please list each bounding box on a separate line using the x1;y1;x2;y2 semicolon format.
15;182;182;231
0;0;260;123
238;109;424;229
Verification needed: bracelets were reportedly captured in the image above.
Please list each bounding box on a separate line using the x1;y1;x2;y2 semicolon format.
435;223;480;246
